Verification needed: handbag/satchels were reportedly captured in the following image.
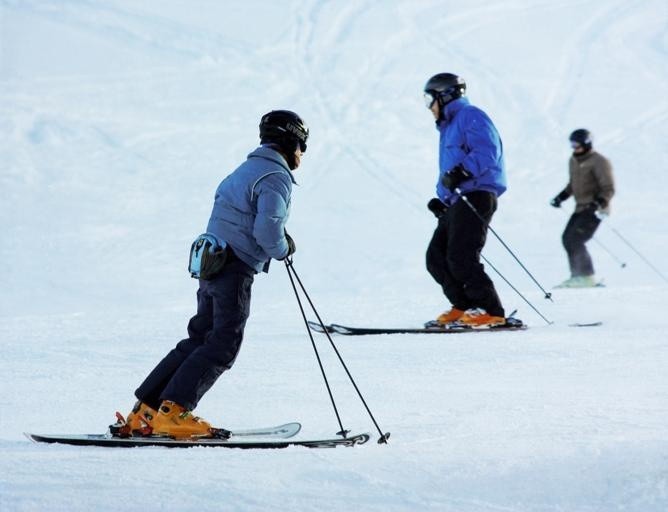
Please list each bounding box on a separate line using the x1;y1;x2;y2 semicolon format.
188;233;229;279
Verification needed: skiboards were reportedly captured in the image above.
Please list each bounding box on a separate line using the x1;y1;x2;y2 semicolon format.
24;421;371;450
307;322;597;336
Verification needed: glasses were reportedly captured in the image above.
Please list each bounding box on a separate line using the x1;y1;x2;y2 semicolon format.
425;86;458;109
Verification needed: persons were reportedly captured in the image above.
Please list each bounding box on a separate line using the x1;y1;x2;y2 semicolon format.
423;72;508;329
550;129;614;278
116;110;310;439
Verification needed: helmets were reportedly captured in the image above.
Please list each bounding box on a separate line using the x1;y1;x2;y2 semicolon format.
259;110;310;171
570;129;592;150
425;72;466;108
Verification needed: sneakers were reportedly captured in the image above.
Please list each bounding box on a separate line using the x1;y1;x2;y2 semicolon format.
561;276;597;288
126;400;215;438
438;307;506;328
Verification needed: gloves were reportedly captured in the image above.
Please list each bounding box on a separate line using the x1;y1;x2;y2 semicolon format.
587;197;606;220
277;228;295;260
427;198;447;218
551;191;570;208
442;163;474;194
